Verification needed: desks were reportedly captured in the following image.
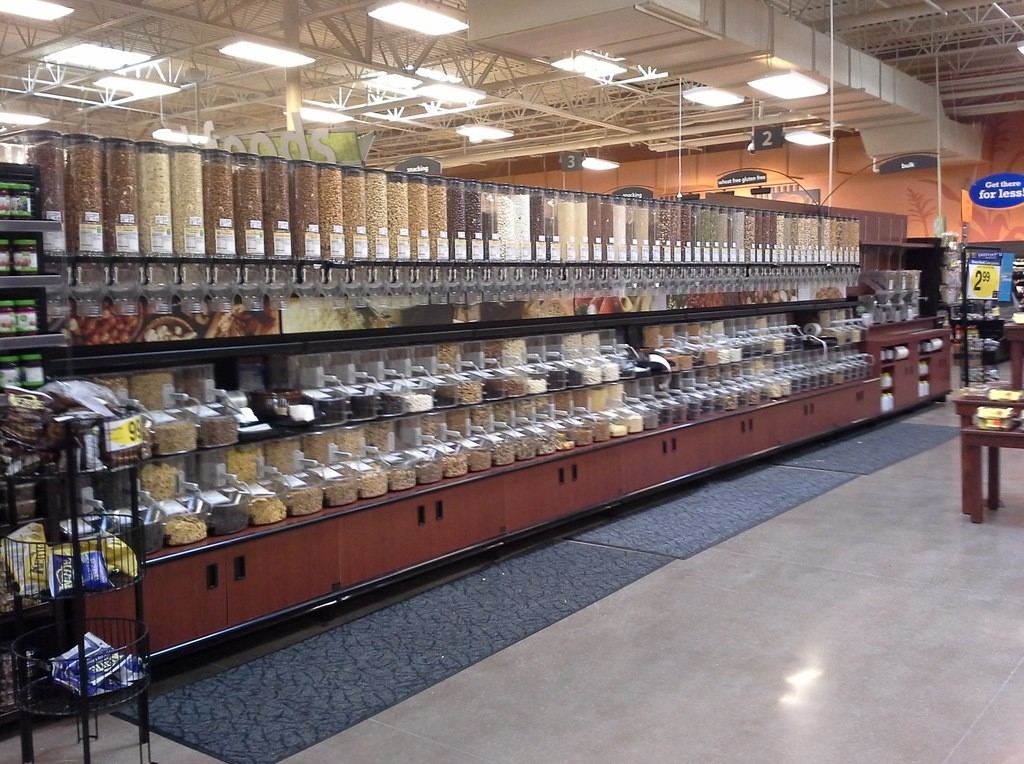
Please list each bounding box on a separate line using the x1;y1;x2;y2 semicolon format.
952;388;1024;514
961;424;1024;523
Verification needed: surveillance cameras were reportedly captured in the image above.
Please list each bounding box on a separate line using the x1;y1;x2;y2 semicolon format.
747;143;755;154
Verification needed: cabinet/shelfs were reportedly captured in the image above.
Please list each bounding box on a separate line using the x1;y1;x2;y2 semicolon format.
1;207;951;733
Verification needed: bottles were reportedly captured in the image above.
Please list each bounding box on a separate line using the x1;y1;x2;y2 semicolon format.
5;130;861;313
